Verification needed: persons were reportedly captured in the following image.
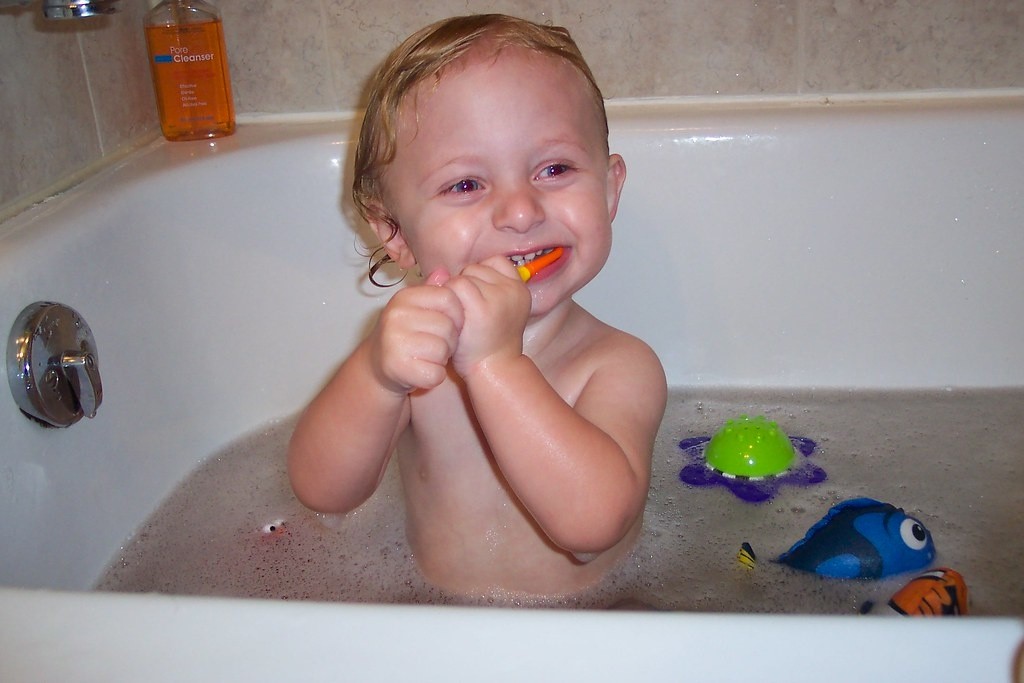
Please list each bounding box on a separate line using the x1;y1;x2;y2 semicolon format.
287;11;668;596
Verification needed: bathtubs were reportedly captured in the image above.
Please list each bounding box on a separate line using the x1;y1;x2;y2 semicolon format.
1;91;1024;682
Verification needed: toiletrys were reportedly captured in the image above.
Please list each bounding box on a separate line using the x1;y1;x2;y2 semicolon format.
142;0;236;143
